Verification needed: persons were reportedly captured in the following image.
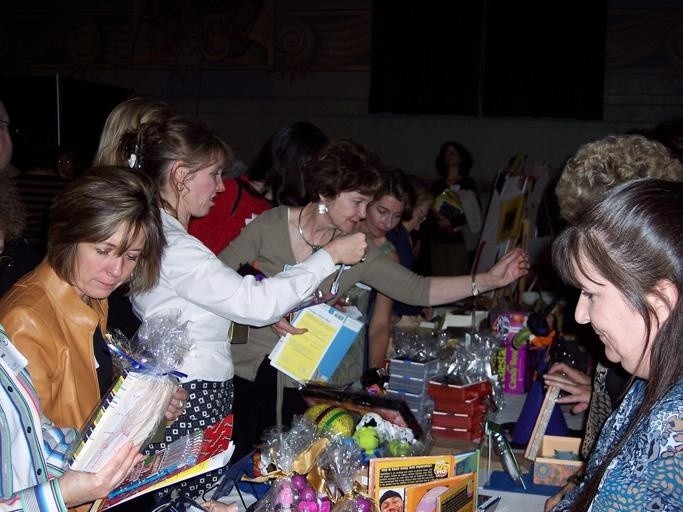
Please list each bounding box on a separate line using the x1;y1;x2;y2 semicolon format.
0;324;190;512
543;134;682;462
188;121;333;255
277;165;415;434
1;165;169;512
0;98;29;302
544;179;683;512
368;180;435;334
216;139;531;469
92;97;368;511
410;140;484;278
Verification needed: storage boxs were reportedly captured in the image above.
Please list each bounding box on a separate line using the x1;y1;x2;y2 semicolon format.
522;384;584;488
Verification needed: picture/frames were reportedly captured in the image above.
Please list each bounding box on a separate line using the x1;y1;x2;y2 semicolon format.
293;384;424;446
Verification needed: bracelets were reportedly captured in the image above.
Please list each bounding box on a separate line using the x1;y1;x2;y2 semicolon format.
470;273;480;296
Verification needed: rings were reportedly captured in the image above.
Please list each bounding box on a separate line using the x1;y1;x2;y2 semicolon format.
176;400;183;410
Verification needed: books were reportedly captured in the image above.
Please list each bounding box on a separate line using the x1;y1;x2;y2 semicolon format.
88;414;235;512
370;447;479;512
267;302;366;387
62;367;182;477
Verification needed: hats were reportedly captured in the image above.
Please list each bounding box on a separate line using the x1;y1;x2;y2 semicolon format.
380;490;402;510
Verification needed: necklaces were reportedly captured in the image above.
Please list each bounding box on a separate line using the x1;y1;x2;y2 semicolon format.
298;206;336;253
